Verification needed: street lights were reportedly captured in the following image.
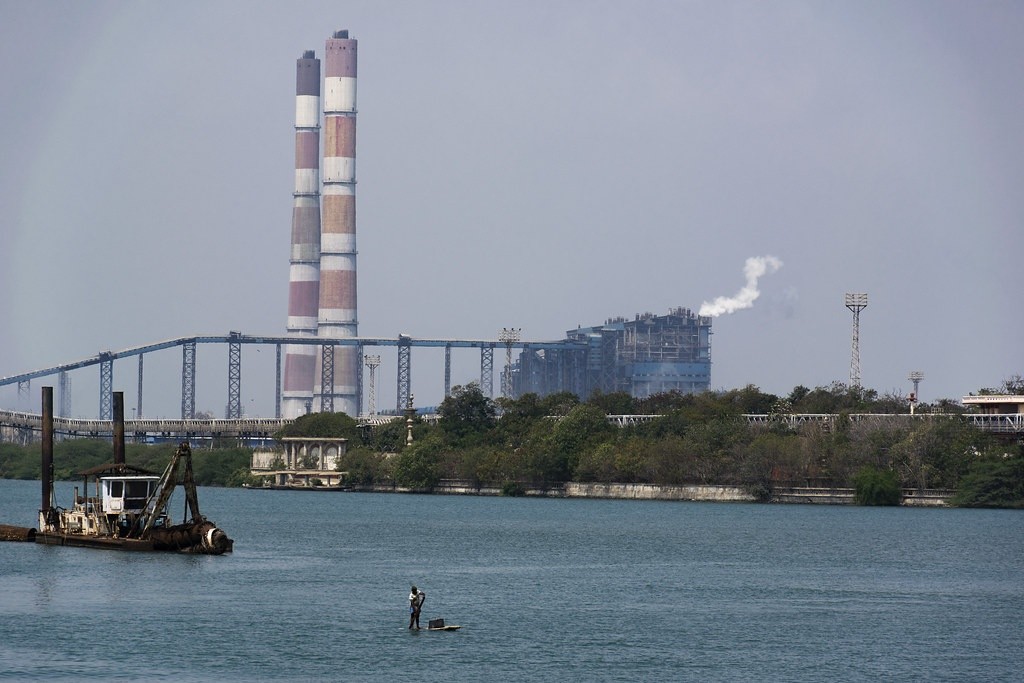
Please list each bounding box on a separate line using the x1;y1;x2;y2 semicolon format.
404;395;417;449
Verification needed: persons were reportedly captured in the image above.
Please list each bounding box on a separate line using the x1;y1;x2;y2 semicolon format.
409;586;425;629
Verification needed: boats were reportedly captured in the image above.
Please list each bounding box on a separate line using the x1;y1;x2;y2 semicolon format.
35;386;233;554
419;625;461;631
